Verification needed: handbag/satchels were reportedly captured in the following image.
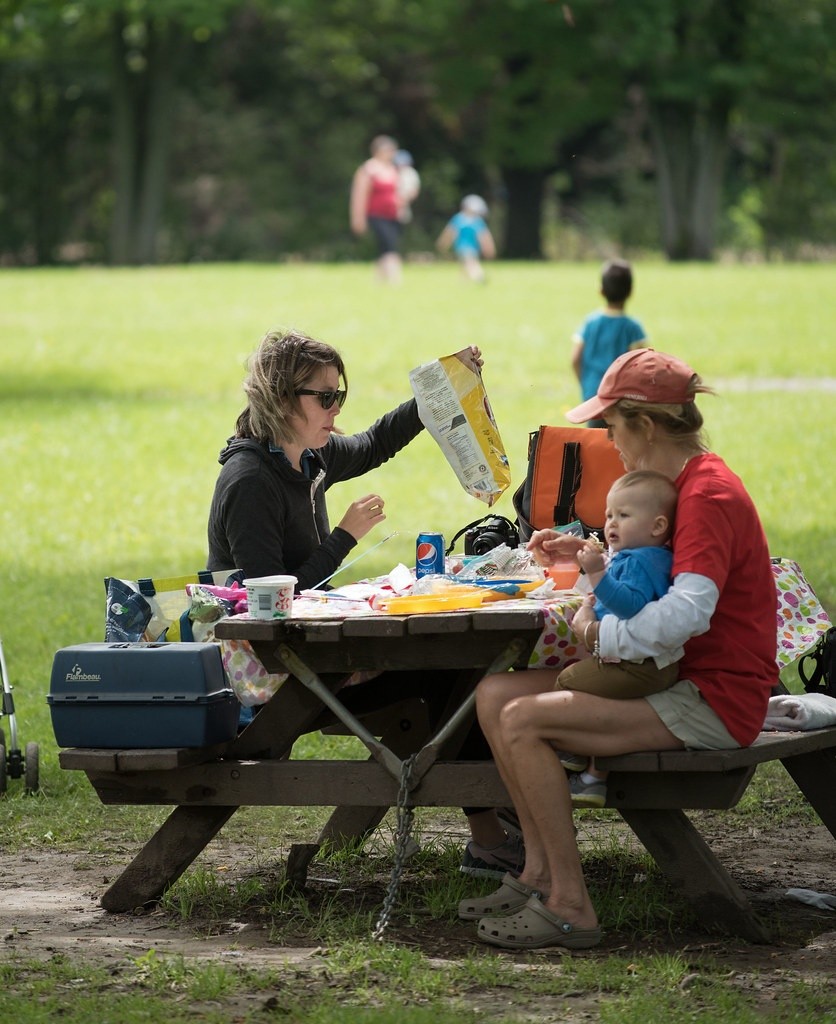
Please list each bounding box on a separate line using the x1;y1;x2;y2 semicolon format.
514;425;629;550
798;624;836;698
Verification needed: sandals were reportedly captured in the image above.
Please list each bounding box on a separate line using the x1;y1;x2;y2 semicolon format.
450;872;547;915
472;895;604;951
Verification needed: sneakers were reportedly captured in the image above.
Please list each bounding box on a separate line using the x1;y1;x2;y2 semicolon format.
458;806;525;876
554;751;609;812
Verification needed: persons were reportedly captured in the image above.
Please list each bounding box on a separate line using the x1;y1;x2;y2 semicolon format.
436;193;497;285
552;470;681;772
571;262;650;429
205;331;526;881
458;348;777;948
348;135;421;285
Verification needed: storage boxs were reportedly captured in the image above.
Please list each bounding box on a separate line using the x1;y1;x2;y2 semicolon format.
43;640;243;750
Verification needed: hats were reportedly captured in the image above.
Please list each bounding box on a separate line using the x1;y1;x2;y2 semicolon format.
565;345;698;427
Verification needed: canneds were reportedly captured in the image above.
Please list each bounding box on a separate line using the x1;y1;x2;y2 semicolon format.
416;532;445;581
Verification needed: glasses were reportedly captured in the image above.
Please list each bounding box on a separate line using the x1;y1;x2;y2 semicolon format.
284;384;348;409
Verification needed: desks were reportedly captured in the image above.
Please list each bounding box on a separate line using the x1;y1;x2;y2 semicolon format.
57;551;835;947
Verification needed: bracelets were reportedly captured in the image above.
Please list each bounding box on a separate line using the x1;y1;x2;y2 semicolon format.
584;621;600;659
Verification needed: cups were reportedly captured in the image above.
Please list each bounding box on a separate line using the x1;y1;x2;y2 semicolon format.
242;576;299;619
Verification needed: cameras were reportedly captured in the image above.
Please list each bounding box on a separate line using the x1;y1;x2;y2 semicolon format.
464;517;520;555
